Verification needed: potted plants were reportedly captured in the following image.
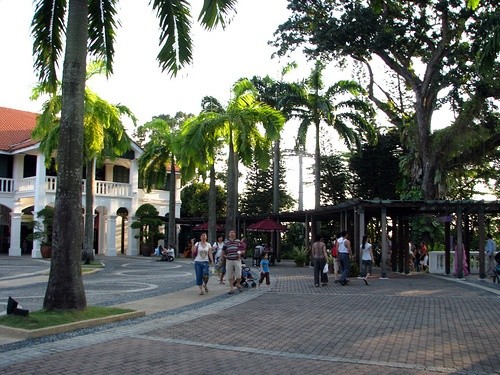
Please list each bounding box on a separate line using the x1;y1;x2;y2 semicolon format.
130;203;163;257
293;244;310;268
25;205;55;258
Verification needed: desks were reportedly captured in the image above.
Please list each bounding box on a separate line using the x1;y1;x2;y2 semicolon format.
263;251;276;265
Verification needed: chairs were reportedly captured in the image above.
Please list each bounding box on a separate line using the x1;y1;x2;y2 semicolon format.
253;248;261;267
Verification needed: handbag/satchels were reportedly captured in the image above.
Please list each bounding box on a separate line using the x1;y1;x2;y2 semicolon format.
191;242;199;258
322;263;328;273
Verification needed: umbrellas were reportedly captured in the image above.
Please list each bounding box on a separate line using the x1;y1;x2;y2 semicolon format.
192;222;225;234
247;217;287;240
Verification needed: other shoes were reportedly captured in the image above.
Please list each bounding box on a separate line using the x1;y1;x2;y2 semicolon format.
363;279;369;285
202;284;209;293
199;291;205;295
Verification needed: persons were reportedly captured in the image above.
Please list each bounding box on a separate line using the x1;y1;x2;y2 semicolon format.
184;241;191;258
158;243;177;262
359;235;374;285
258;252;271;286
190;233;214;294
311;230;353;286
372;234;429;272
252;240;272;264
484;233;500;282
23;239;28;255
210;231;255;294
452;236;470;277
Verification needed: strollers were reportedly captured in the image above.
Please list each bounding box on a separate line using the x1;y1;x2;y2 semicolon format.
234;260;257;288
154;245;175;262
